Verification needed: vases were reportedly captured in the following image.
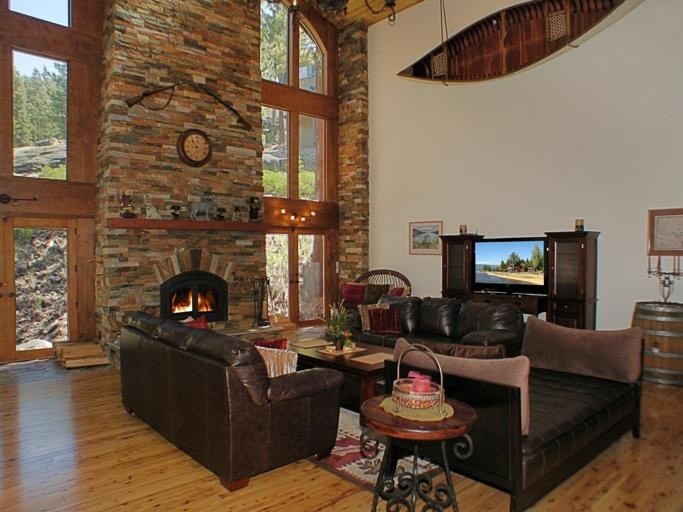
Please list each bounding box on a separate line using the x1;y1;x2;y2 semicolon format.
334;338;345;351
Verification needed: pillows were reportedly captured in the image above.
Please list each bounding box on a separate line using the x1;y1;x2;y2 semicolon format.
182;313;210;330
390;337;531;438
252;343;299;379
252;336;288;354
518;315;644;387
340;281;410;335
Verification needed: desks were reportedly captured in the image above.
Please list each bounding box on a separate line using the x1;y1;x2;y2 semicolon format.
360;392;479;512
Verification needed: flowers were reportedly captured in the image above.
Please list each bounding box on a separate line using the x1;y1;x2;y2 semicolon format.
320;296;362;343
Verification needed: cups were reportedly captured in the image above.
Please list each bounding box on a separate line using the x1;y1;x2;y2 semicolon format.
574;218;584;232
458;224;467;234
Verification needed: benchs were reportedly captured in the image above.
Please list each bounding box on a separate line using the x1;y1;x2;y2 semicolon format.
383;315;646;511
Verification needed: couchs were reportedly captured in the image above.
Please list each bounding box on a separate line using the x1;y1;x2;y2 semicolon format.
354;293;524;359
120;308;345;492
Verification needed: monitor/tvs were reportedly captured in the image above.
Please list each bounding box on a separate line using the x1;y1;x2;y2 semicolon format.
472;237;548;297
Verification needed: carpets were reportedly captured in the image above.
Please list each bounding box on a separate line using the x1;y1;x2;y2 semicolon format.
307;406;444;498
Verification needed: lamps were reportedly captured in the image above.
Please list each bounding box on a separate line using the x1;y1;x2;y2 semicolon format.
263;0;397;23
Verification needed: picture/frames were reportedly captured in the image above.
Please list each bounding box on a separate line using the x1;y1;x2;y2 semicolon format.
645;207;683;256
408;220;444;256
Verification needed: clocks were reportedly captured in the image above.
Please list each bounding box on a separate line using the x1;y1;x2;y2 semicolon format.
177;127;214;170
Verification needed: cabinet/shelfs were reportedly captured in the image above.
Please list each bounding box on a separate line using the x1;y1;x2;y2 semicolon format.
472;293;547;318
543;230;601;330
437;233;485;300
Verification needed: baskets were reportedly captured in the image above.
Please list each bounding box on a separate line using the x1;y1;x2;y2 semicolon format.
392;343;444;410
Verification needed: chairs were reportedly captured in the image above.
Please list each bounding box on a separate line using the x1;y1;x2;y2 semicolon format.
338;269;412;319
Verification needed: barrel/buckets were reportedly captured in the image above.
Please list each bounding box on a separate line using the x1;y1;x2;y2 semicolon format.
630;301;682;387
